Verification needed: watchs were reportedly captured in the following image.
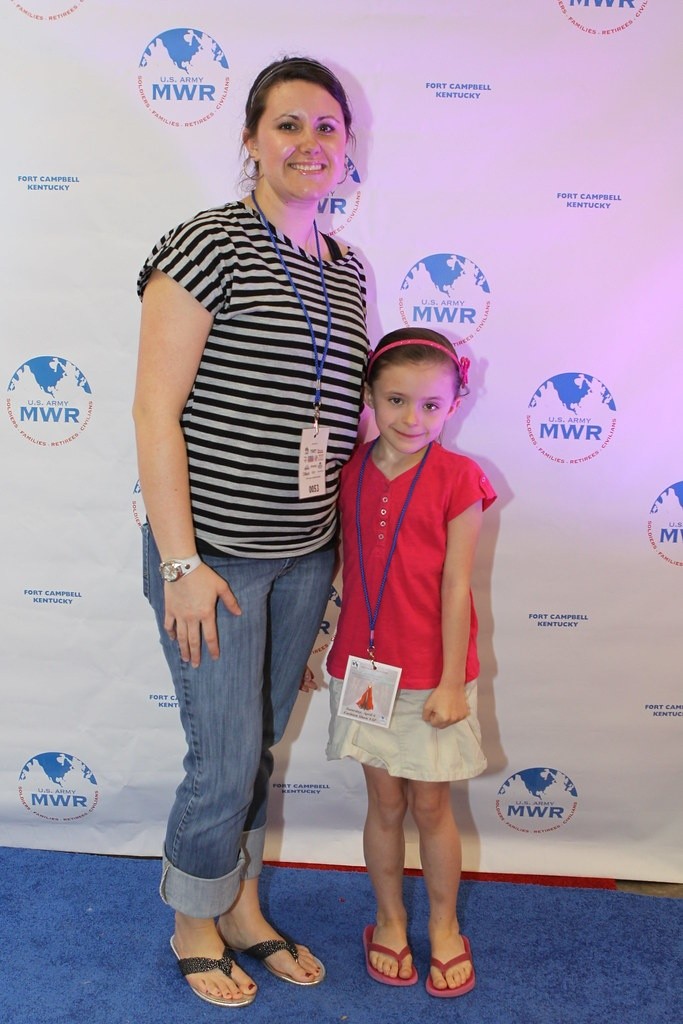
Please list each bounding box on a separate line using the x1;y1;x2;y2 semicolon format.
159;553;207;582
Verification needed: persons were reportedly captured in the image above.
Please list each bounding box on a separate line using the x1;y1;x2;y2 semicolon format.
299;329;498;998
134;60;369;1005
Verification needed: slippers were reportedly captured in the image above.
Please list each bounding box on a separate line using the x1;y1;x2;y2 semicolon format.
226;928;325;986
363;924;418;986
170;934;256;1008
426;935;475;998
